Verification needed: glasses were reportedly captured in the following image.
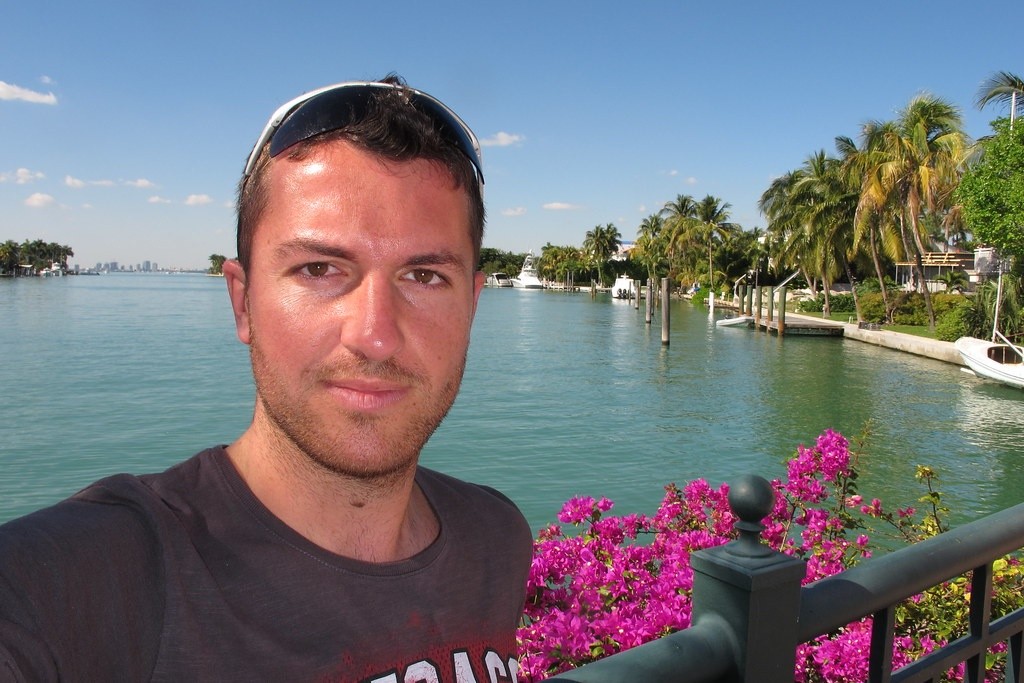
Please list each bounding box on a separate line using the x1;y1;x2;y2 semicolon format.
236;81;486;264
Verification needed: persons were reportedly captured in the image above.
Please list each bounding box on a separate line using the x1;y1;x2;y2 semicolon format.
0;71;532;683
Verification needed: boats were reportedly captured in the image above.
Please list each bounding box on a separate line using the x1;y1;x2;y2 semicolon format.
953;333;1024;390
509;250;543;290
715;315;754;328
611;269;636;299
486;272;513;287
